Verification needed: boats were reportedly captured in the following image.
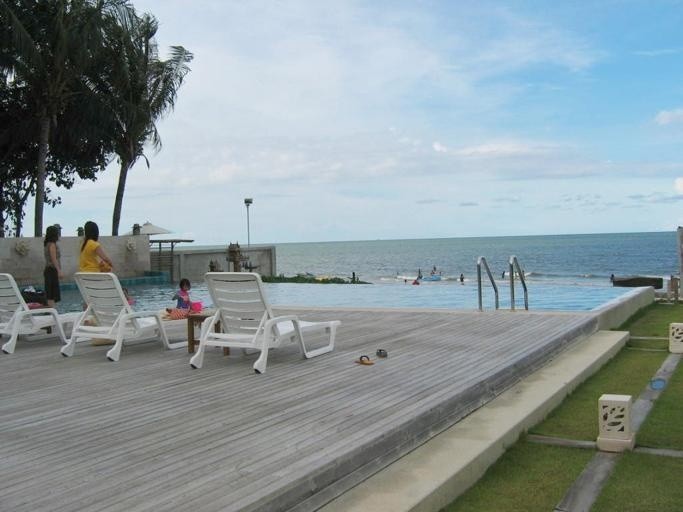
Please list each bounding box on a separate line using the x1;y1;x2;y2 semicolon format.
416;274;441;281
501;271;533;278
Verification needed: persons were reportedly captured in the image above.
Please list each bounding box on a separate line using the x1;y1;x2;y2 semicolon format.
78;220;112;325
609;273;615;282
171;278;190;308
415;265;442;278
121;287;137;304
42;225;64;314
459;274;464;282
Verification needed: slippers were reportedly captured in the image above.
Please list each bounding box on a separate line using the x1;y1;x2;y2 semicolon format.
376;349;387;358
354;354;375;366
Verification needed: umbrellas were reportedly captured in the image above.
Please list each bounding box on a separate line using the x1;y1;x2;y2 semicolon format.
121;220;175;239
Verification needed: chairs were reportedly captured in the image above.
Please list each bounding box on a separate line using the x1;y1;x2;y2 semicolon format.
60;271;200;361
0;273;95;354
189;272;342;375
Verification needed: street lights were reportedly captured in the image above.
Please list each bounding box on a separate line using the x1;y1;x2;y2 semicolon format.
243;197;253;247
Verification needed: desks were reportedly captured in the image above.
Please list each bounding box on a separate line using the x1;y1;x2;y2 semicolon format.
187;314;230;356
